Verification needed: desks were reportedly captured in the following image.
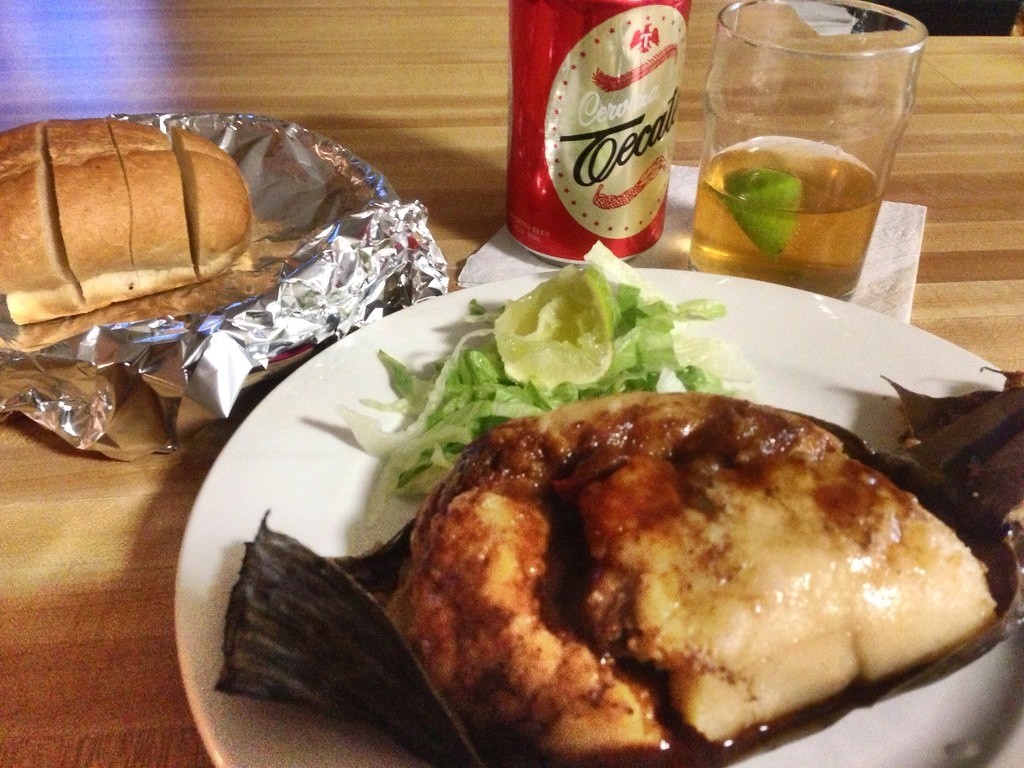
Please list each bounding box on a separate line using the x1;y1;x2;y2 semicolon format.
0;0;1024;768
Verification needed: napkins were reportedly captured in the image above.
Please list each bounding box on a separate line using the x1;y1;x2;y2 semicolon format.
458;164;927;325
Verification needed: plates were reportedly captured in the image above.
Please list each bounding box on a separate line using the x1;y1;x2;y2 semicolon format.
175;266;1024;768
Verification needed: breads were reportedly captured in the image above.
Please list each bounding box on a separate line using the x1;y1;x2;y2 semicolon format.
0;119;253;325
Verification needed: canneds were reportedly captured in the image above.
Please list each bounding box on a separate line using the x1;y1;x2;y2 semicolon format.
506;0;693;269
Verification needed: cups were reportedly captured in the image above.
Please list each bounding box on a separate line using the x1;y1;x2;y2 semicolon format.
688;0;928;303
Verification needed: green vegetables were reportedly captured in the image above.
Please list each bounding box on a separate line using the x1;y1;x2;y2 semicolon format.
340;263;749;524
